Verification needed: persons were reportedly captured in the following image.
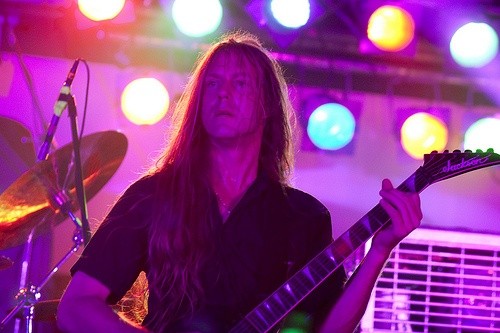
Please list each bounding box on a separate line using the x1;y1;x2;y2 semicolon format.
55;34;422;333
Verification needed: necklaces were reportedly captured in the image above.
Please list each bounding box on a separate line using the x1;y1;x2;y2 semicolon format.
215;190;233;214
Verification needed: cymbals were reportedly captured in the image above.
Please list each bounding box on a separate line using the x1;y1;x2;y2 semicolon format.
0;130;129;250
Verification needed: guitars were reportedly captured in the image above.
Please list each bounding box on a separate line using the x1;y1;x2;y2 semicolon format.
224;148;500;333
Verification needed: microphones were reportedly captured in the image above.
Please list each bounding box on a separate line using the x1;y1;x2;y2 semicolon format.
37;59;80;159
